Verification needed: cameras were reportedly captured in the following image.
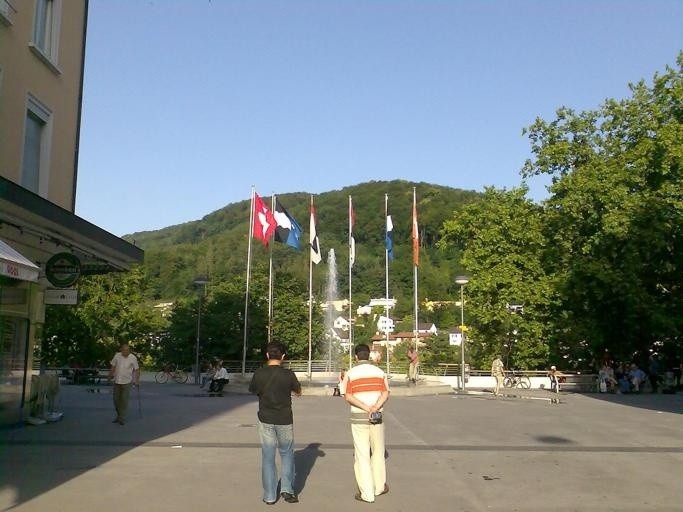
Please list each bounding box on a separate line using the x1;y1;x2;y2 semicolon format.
372;413;383;419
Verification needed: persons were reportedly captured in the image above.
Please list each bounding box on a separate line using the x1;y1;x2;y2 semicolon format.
345;344;390;503
407;347;420;382
207;360;229;392
546;366;566;383
107;342;141;425
491;355;505;396
338;369;346;383
249;340;302;505
199;363;216;389
597;351;682;395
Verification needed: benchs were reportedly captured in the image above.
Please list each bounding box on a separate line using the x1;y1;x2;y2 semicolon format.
57;365;114;383
551;372;600;394
607;373;653;393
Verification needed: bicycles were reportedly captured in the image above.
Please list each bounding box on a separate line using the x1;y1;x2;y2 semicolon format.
503;365;532;389
154;357;190;385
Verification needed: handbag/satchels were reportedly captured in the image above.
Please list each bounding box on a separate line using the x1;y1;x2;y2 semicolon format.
599;378;607;393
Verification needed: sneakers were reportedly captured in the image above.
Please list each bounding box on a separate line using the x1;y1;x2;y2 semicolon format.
262;498;276;505
280;492;299;504
380;484;389;493
354;493;374;503
112;420;126;426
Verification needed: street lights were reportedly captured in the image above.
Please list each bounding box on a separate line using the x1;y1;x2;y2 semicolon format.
455;274;472;394
192;278;210;386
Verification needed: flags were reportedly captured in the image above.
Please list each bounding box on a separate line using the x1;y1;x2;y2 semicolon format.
310;203;322;265
349;200;357;270
412;190;421;267
385;196;394;259
252;192;279;250
273;195;304;252
